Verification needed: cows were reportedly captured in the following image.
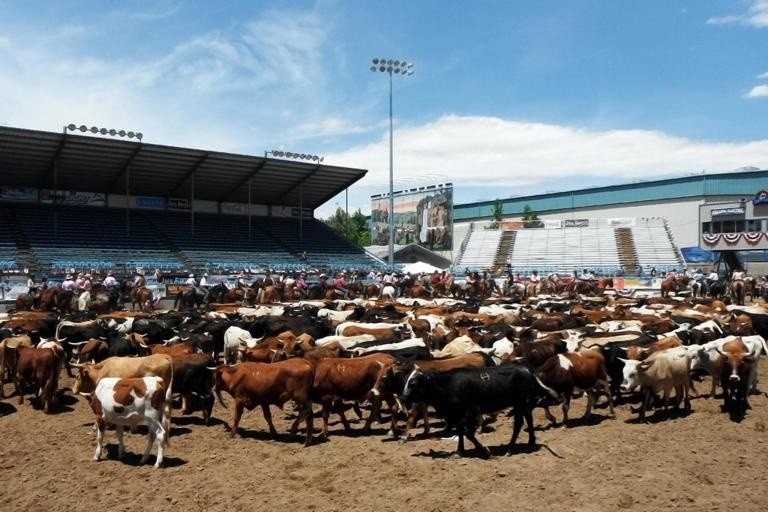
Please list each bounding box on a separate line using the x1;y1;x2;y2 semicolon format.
400;364;560;460
6;342;59;414
77;376;171;470
685;335;768;399
617;345;699;421
171;279;618;302
172;353;227;422
68;353;174;436
308;353;406;438
531;348;617;431
400;351;498;433
206;358;316;446
1;297;768;396
714;336;756;413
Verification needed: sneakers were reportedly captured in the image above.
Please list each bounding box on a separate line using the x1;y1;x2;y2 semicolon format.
203;300;208;304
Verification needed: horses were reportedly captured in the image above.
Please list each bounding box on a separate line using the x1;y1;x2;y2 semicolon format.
659;275;748;304
14;277;156;310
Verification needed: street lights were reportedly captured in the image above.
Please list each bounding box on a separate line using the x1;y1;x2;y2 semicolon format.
369;57;415;264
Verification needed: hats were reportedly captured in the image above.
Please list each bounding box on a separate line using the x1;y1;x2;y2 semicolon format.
108;270;113;275
189;274;194;277
204;273;208;277
65;272;91;279
136;267;145;276
377;270;392;276
421;271;427;276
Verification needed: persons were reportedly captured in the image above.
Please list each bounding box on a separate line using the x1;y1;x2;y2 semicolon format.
616;264;768;301
464;262;601;286
185;269;450;300
26;266;147;294
